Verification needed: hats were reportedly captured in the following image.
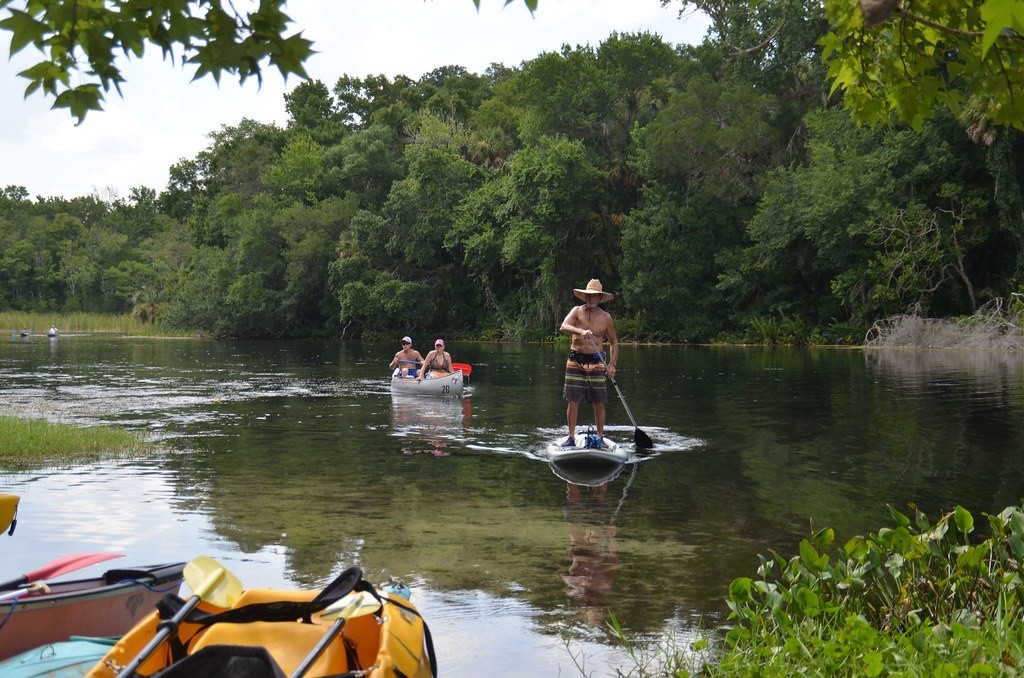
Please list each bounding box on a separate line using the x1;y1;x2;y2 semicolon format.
401;337;411;343
574;279;614;303
435;339;444;346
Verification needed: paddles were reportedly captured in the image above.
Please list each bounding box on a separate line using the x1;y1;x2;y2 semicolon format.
395;360;472;377
0;552;128;594
290;591;383;678
587;333;653;449
114;554;245;678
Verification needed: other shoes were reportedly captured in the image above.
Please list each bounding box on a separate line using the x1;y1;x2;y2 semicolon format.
596;437;610;450
561;438;576;447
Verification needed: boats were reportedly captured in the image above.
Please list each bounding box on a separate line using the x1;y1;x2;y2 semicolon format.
0;562;439;678
391;366;465;400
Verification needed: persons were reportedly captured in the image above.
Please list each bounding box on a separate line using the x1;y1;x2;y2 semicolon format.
389;336;453;381
560;484;618;627
50;325;57;335
559;279;618;448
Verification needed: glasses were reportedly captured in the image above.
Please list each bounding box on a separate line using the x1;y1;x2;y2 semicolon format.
402;342;409;345
